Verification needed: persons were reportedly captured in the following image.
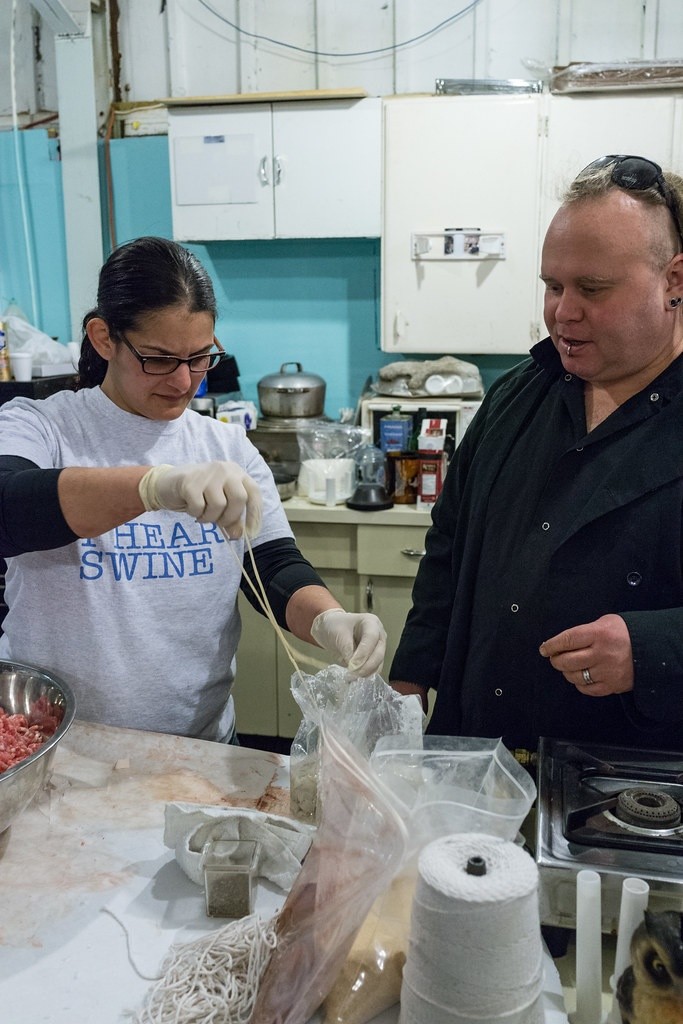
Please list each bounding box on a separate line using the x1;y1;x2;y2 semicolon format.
366;156;683;757
0;238;386;748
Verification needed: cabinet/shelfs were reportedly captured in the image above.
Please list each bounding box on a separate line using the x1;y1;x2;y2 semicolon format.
166;89;683;355
236;494;433;750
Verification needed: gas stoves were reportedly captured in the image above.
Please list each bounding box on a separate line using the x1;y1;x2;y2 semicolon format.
535;734;683;934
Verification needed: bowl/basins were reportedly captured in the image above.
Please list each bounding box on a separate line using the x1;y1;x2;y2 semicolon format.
0;658;77;834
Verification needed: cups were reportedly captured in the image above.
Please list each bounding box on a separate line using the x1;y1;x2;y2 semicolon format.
68;342;80;370
8;352;32;381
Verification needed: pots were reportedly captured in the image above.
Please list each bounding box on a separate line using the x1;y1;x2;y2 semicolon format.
258;362;326;417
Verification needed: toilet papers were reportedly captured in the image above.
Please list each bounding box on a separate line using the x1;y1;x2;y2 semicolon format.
397;832;571;1024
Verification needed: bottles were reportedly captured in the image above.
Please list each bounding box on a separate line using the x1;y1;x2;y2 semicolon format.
355;443;389;491
3;298;30;323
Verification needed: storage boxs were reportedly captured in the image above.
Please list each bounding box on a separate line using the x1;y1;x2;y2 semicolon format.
198;839;262;917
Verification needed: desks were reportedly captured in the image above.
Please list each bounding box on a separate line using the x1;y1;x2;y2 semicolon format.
0;719;290;1024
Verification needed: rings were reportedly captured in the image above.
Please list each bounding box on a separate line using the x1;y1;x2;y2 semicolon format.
582;668;594;684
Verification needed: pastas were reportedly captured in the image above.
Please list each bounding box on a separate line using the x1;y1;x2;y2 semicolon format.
98;902;282;1024
218;522;307;685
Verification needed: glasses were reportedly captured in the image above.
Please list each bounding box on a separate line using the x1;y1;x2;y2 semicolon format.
576;155;683;255
110;326;226;373
